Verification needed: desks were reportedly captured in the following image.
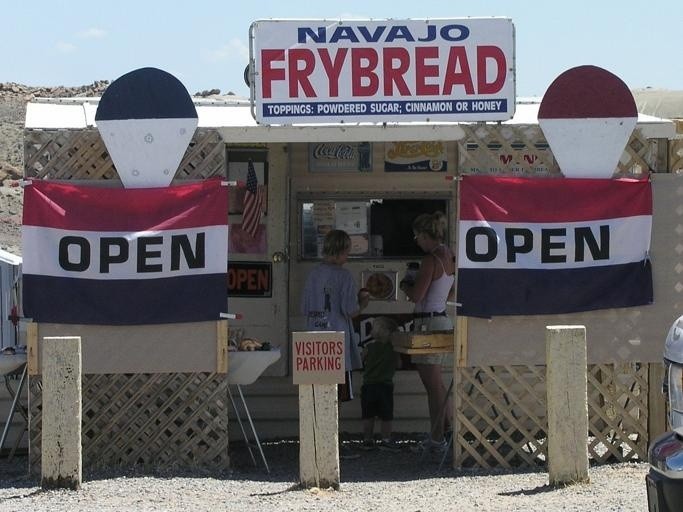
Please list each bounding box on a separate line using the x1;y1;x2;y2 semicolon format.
0;350;29;455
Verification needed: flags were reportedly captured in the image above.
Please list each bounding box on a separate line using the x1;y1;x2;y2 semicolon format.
452;171;655;321
17;178;231;327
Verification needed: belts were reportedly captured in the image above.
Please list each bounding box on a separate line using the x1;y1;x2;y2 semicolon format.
412;311;448;319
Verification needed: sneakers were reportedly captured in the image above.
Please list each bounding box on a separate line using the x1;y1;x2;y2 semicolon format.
339;434;449;461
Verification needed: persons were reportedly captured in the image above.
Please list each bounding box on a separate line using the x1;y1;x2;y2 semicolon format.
358;314;404;452
300;227;371;459
400;209;455;465
